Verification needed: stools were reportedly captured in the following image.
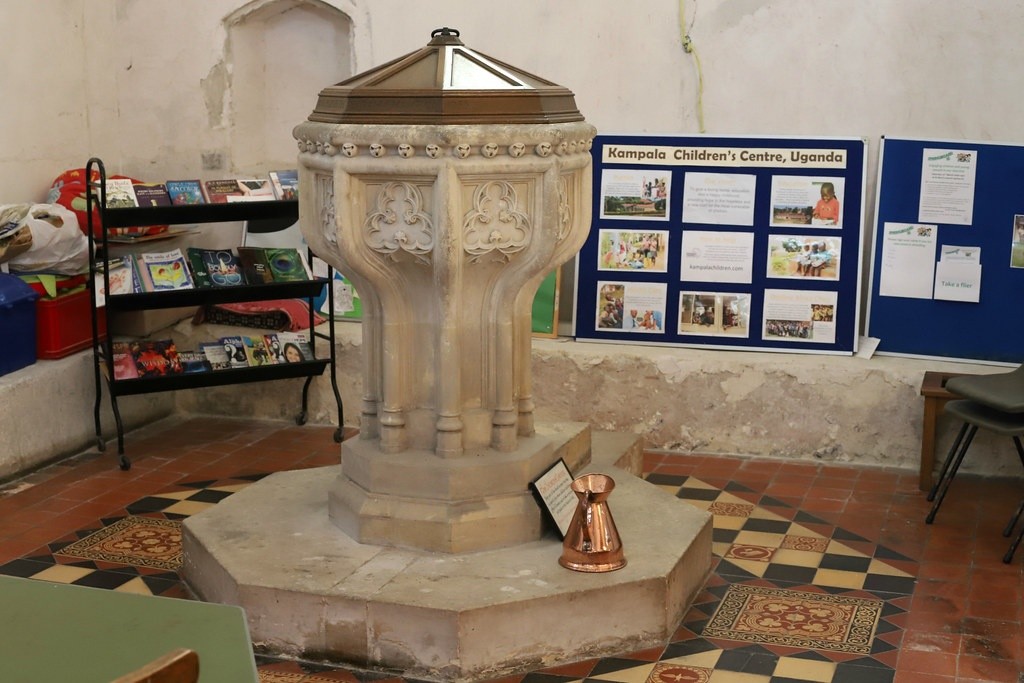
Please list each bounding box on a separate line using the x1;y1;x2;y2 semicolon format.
925;362;1024;563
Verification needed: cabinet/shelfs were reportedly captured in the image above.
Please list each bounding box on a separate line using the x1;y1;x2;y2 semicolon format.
85;158;344;470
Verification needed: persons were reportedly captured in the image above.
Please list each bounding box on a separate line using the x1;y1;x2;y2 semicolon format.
284;343;305;361
252;342;267;365
598;176;839;340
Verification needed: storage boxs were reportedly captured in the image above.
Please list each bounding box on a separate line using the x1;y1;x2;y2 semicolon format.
0;271;41;377
27;270;106;360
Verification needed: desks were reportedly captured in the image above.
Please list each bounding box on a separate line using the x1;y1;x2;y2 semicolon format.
917;371;982;489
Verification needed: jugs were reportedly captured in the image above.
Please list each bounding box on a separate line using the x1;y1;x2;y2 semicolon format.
558;472;628;572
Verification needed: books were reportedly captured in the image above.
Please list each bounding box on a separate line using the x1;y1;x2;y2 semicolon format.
94;169;316;377
